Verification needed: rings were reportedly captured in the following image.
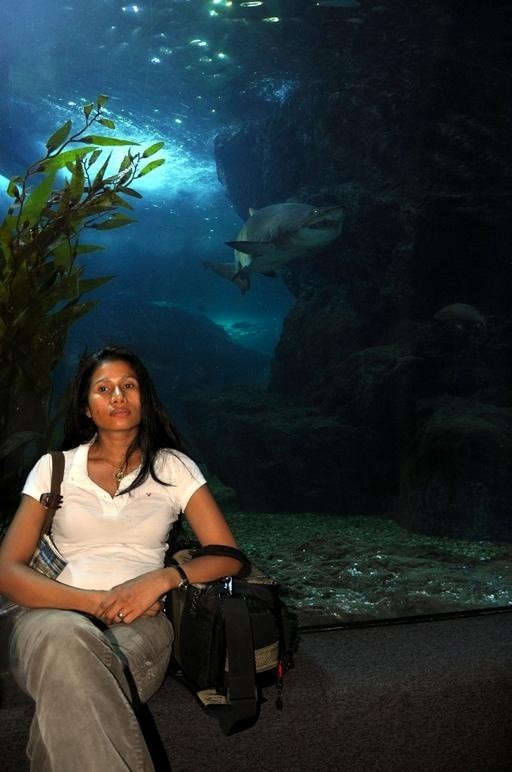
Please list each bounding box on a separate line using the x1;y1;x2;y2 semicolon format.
117;611;126;619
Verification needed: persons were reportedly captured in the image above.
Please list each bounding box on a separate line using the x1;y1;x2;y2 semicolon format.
0;346;242;772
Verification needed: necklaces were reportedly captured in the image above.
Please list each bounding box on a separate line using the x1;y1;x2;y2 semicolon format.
114;466;126;490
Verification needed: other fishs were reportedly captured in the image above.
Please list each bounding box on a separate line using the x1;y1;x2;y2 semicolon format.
433;302;488;339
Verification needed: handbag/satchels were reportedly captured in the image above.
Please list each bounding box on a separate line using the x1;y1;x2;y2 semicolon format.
159;542;302;739
0;450;68;628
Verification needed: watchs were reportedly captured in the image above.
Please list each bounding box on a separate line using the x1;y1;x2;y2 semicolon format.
171;564;191;592
